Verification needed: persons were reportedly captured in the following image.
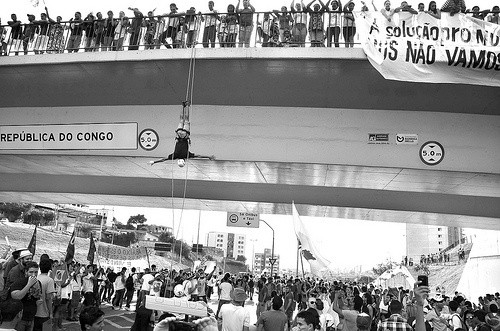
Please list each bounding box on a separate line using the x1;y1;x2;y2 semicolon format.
0;247;500;331
0;0;500;56
147;101;217;166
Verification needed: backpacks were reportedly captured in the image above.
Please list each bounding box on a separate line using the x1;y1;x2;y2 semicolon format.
451;314;469;331
124;273;135;289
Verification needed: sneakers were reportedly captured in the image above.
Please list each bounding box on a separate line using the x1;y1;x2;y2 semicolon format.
182;101;191;107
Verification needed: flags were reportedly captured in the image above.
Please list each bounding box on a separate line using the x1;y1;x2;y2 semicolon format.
64;231;75;262
27;226;37;260
87;235;96;264
30;0;39;7
292;205;333;279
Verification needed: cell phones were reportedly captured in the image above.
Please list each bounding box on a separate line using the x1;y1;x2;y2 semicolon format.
418;275;428;287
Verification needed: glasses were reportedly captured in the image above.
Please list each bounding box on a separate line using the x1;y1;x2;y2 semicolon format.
466;315;475;319
310;301;316;304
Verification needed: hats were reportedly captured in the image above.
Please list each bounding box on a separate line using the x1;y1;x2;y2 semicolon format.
18;250;33;262
433;294;444;301
390;300;402;314
356;316;370;331
229;288;249;301
27;13;35;19
173;284;184;296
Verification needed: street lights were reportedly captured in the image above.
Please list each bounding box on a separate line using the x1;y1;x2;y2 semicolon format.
260;219;276;277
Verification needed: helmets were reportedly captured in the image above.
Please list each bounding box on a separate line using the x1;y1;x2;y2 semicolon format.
178;159;185;167
